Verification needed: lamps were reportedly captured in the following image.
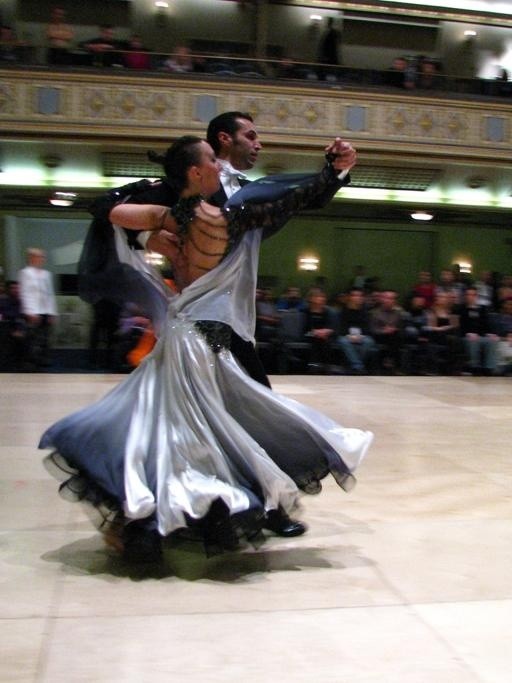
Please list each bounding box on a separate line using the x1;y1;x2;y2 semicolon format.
410;213;433;220
49;195;75;207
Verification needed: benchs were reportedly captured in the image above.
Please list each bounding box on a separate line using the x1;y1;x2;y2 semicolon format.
255;306;499;375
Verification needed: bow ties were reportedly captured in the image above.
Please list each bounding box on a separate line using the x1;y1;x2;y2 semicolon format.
222;167;247;179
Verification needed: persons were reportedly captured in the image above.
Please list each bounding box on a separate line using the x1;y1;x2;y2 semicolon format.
39;136;376;579
1;246;57;373
46;7;342;83
253;262;511;377
2;22;34;69
413;60;443;89
85;263;178;372
101;109;357;558
382;58;419;92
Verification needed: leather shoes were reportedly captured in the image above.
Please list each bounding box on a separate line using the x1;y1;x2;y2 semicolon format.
124;524;164;560
204;524;246;558
258;507;305;535
